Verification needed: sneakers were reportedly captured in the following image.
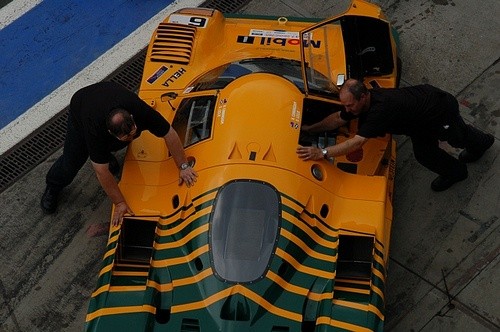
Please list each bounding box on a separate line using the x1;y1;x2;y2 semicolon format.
109;154;119;176
41;187;59;213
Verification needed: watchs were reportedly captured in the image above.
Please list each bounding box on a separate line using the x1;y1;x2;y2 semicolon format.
179;163;190;169
322;148;328;159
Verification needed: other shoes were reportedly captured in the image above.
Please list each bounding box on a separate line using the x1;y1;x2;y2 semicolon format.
459;132;495;163
431;163;469;193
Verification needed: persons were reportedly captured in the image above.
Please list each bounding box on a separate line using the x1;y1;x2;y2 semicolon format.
297;78;494;191
40;82;198;228
